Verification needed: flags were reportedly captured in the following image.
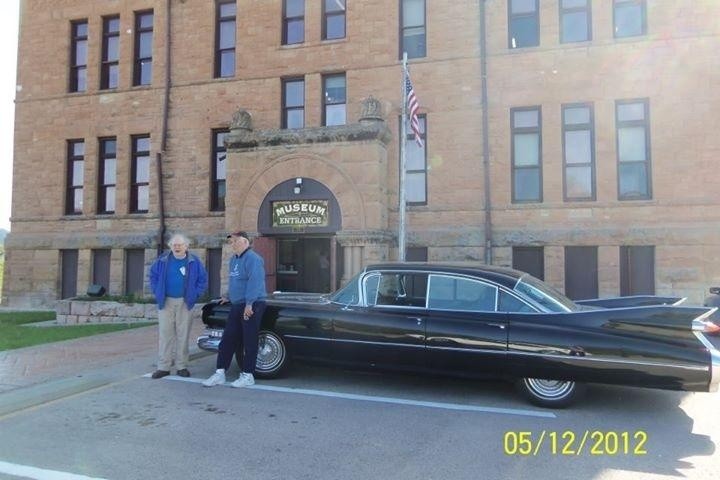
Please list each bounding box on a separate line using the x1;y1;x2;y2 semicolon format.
406;74;424;148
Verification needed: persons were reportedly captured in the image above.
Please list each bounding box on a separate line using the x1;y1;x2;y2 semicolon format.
319;249;329;292
201;231;267;388
148;233;208;379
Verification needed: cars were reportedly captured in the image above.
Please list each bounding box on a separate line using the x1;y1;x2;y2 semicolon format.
197;262;720;409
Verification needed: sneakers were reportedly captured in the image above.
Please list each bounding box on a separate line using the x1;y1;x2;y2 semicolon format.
153;370;171;379
201;369;227;387
230;372;255;387
178;369;190;377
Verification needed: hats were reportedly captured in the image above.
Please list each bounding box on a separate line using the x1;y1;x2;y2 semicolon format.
227;231;250;243
